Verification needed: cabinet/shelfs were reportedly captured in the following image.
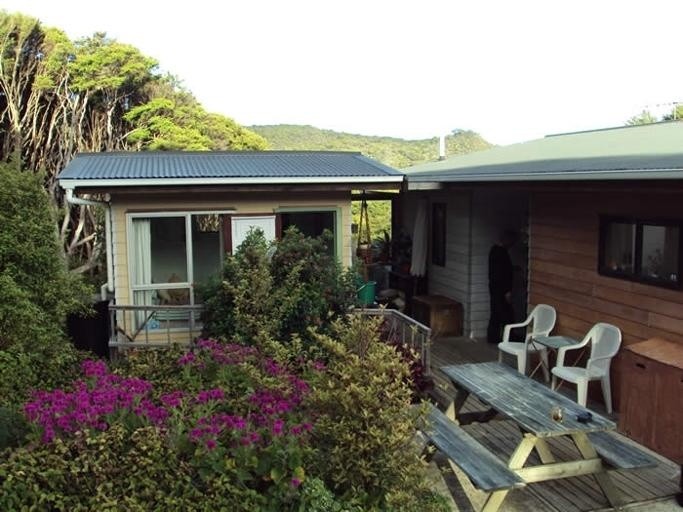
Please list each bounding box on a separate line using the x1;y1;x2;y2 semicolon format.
618;338;683;465
388;269;427;316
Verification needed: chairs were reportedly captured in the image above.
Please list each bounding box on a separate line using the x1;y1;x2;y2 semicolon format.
497;303;622;414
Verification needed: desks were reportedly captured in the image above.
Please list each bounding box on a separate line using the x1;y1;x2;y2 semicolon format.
432;357;624;512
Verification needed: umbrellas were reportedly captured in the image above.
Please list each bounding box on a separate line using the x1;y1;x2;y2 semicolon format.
408;193;428;296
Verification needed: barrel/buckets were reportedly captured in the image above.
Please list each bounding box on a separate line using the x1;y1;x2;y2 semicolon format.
356;281;376;305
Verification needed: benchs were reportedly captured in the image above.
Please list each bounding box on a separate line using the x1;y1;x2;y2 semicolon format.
412;294;462;337
409;402;660;510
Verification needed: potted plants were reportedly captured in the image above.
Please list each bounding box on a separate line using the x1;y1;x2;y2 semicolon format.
371;230;392;261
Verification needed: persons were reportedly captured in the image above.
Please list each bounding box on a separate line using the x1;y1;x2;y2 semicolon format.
487;235;523;344
356;249;369;305
363;257;390;294
388;260;415;303
